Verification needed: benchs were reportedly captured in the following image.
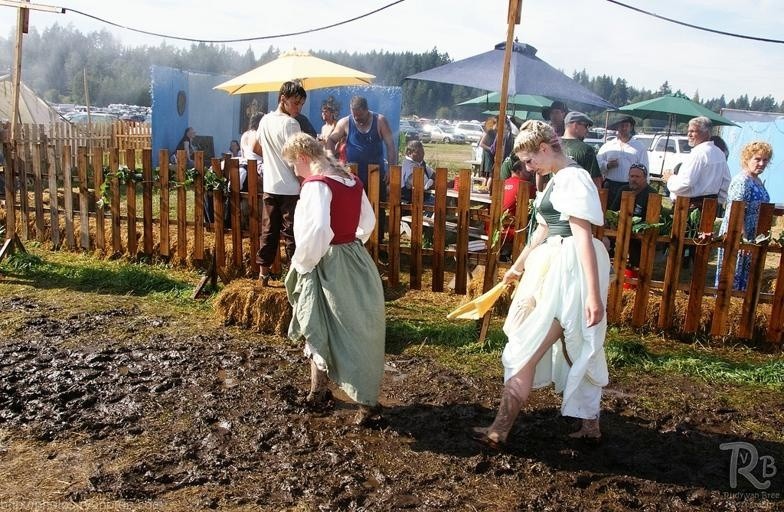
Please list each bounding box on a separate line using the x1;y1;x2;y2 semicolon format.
401;214;488;241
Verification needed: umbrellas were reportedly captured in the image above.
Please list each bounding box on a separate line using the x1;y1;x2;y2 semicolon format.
212;46;377;97
454;93;572;113
404;35;618;110
607;90;742;194
481;110;549;122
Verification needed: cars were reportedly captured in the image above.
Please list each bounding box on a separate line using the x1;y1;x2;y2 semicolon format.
47;101;150;127
399;118;486;144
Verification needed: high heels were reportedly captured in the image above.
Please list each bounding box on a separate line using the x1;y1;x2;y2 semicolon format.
349;404;384;427
295;391;333;408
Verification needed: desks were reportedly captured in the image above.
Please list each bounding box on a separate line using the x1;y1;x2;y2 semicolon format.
424;185;492;229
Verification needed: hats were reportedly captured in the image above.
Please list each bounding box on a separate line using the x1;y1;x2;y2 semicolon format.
548;100;566;113
564;111;594;126
606;115;636;130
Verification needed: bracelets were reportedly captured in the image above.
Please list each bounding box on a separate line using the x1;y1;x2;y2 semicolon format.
511;265;523;275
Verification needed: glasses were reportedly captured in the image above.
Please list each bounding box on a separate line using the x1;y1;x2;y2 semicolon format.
631;163;645;170
578;122;590;128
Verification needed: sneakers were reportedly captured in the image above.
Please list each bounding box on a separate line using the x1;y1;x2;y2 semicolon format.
258;274;270;286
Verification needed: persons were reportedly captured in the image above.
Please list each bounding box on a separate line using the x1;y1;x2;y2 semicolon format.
402;140;436;217
602;162;663;267
227;140;241;157
176;127;196;168
240;112;266;178
667;115;732;269
280;132;386;426
714;141;774;299
534;111;604;192
541;101;571;137
480;115;498;192
315;103;338;158
325;96;394;257
253;81;307;286
471;120;611;454
595;116;650;209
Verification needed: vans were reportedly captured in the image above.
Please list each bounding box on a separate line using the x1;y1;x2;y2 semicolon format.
634;133;694;177
